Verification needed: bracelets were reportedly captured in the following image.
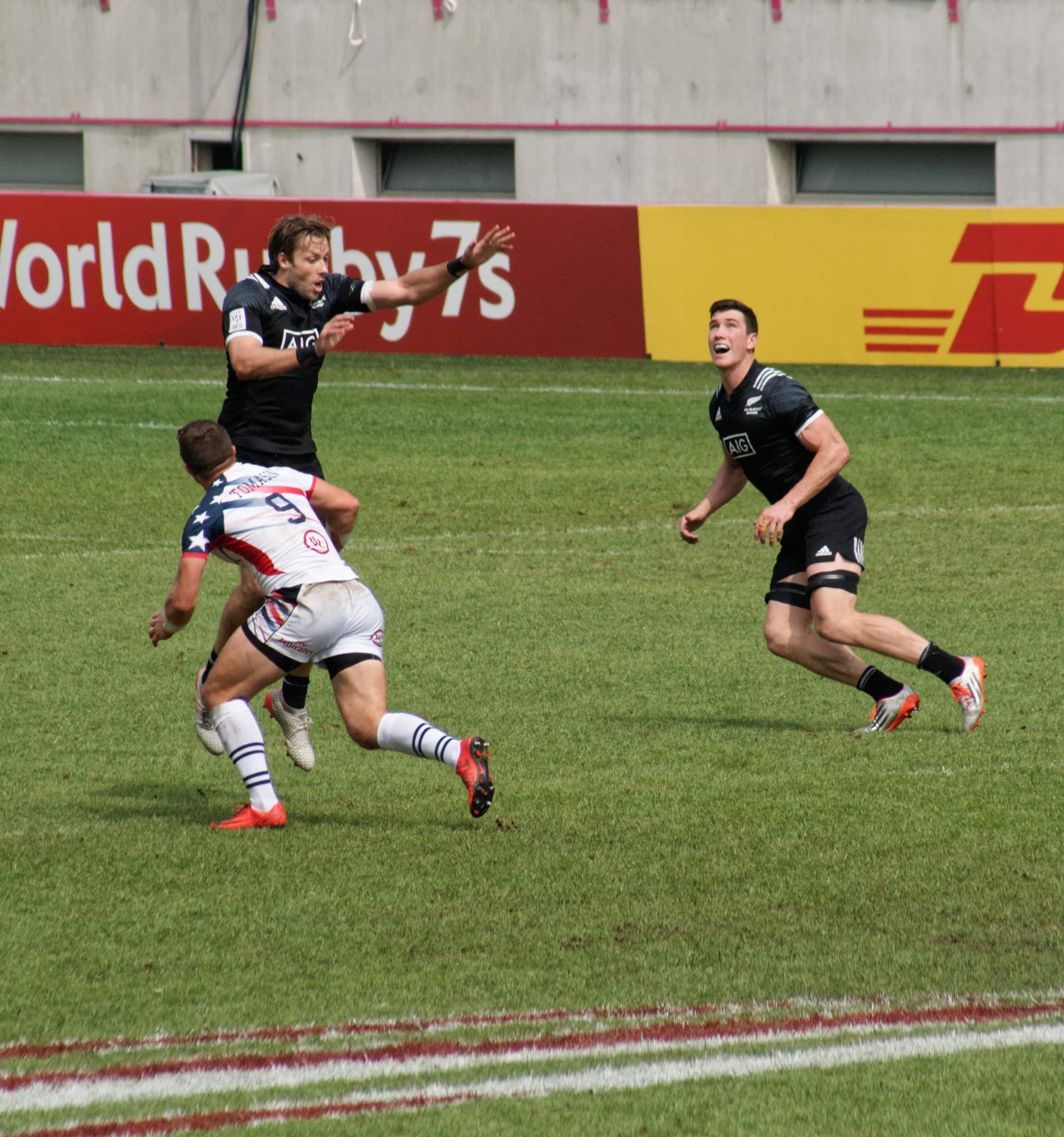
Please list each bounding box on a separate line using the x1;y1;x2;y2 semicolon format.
296;345;319;366
162;612;186;634
446;256;474;278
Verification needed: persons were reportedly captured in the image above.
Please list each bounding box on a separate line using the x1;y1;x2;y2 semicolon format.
187;218;513;769
146;422;496;831
677;299;988;737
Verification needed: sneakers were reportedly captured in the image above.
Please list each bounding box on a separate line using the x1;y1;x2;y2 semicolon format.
455;735;495;818
949;656;988;735
263;688;315;772
851;683;919;735
194;666;225;755
210;802;285;829
